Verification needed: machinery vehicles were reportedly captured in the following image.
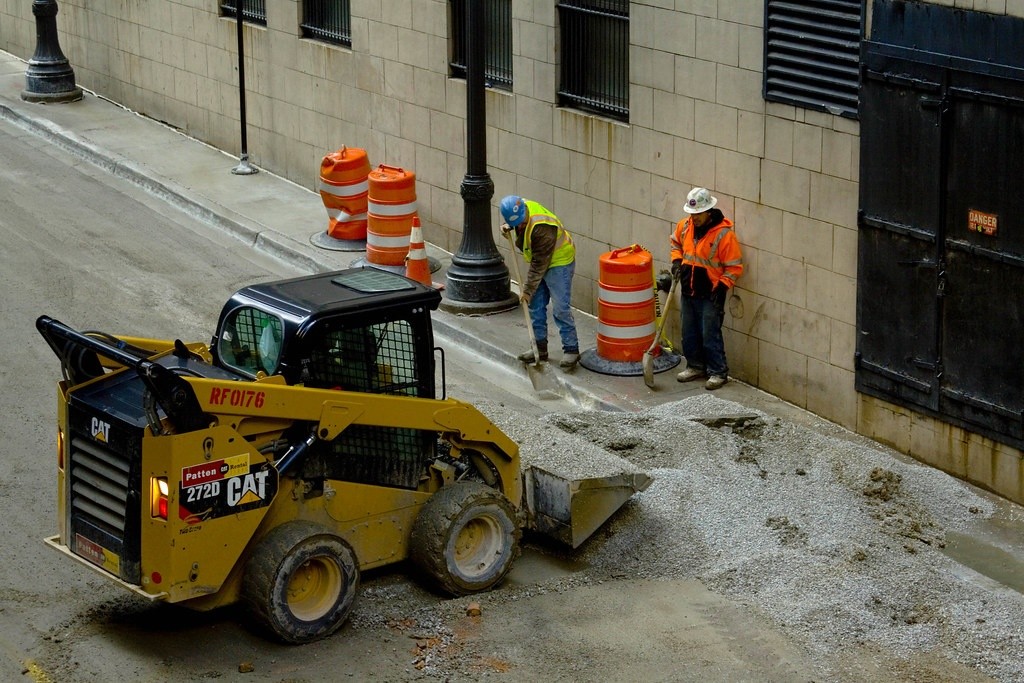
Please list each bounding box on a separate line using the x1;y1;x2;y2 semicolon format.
35;266;654;646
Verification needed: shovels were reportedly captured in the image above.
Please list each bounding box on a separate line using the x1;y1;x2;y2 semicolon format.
506;231;565;400
642;279;677;388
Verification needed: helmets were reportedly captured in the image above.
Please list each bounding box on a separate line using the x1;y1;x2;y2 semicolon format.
500;196;526;226
683;187;717;213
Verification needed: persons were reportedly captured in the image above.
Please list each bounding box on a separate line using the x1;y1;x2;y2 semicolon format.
670;188;744;390
499;196;582;369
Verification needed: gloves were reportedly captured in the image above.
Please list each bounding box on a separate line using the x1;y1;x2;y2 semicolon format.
670;260;682;278
711;282;728;309
519;293;531;306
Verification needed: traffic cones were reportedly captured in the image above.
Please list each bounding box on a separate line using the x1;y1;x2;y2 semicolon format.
405;216;444;290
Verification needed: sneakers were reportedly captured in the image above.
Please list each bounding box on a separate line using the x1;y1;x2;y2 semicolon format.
705;376;728;390
677;367;705;382
559;353;581;367
518;349;548;363
